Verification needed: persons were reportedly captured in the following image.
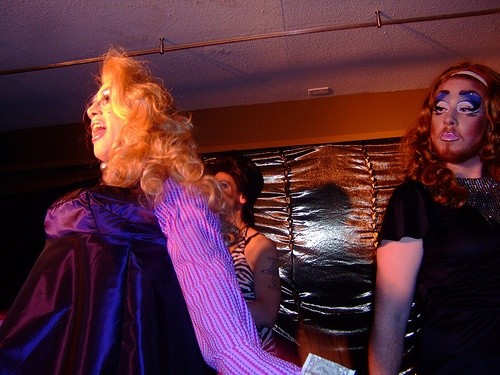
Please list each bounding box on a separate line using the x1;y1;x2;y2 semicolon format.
0;48;308;374
367;62;499;375
208;149;284;358
